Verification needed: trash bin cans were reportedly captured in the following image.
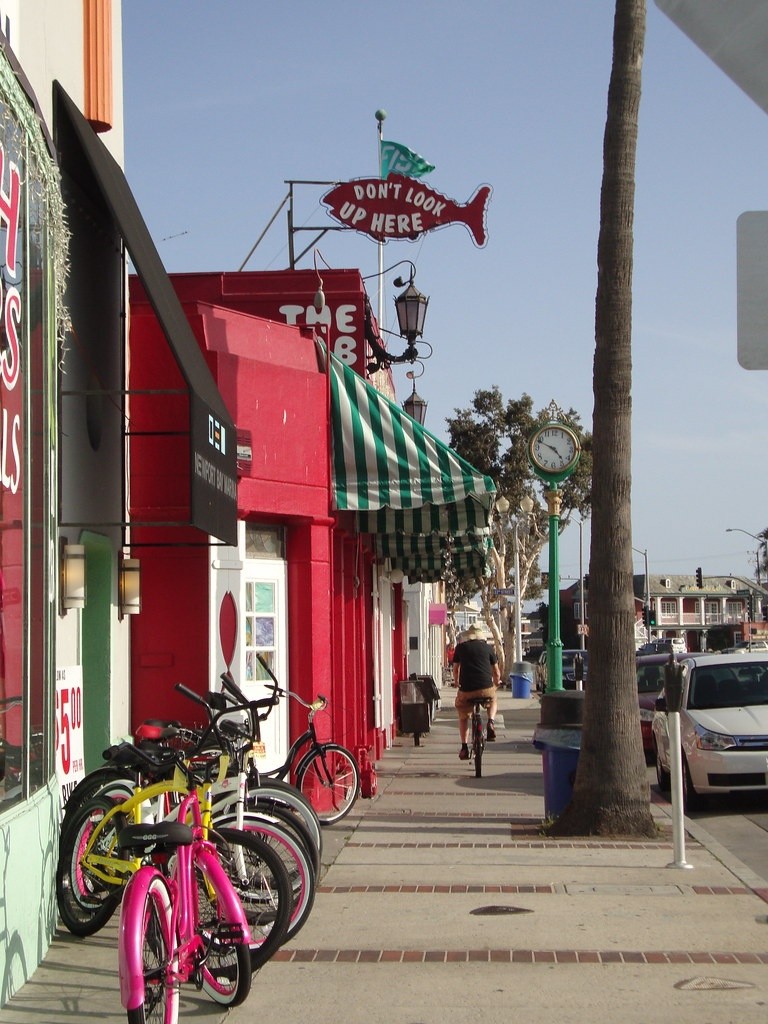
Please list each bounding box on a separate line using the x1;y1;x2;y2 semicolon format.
509;660;533;700
533;720;582;821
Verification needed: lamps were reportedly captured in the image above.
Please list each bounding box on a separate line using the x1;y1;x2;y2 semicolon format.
58;535;87;619
118;550;141;623
364;265;433;426
387;568;404;583
314;247;332;309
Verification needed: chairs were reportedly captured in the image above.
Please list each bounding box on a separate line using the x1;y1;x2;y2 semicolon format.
638;667;665;687
695;674;743;701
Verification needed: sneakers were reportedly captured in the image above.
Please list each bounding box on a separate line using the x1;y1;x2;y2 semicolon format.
487;722;496;739
459;750;469;759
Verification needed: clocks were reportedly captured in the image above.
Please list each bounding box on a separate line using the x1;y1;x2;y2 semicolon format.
521;398;582;483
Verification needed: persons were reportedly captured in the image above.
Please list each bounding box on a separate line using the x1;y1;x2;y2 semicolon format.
447;643;454;686
452;623;500;759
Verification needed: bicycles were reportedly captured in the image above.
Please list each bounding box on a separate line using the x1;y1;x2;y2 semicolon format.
450;681;507;778
57;654;362;1024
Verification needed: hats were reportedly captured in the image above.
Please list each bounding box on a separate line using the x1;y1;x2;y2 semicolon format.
461;623;487;641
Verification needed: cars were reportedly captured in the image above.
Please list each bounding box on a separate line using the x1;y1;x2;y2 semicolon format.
652;652;768;802
634;654;721;764
534;648;589;691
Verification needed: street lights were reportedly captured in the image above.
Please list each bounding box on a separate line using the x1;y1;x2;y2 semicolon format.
726;528;768;577
496;493;535;662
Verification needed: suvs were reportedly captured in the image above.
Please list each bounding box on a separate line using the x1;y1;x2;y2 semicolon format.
722;640;768;654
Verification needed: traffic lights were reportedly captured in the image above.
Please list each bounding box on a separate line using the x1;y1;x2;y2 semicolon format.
649;610;656;626
642;606;647;626
696;567;702;588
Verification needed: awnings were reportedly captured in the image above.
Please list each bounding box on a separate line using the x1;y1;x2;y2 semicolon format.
321;342;496;587
52;79;238;546
